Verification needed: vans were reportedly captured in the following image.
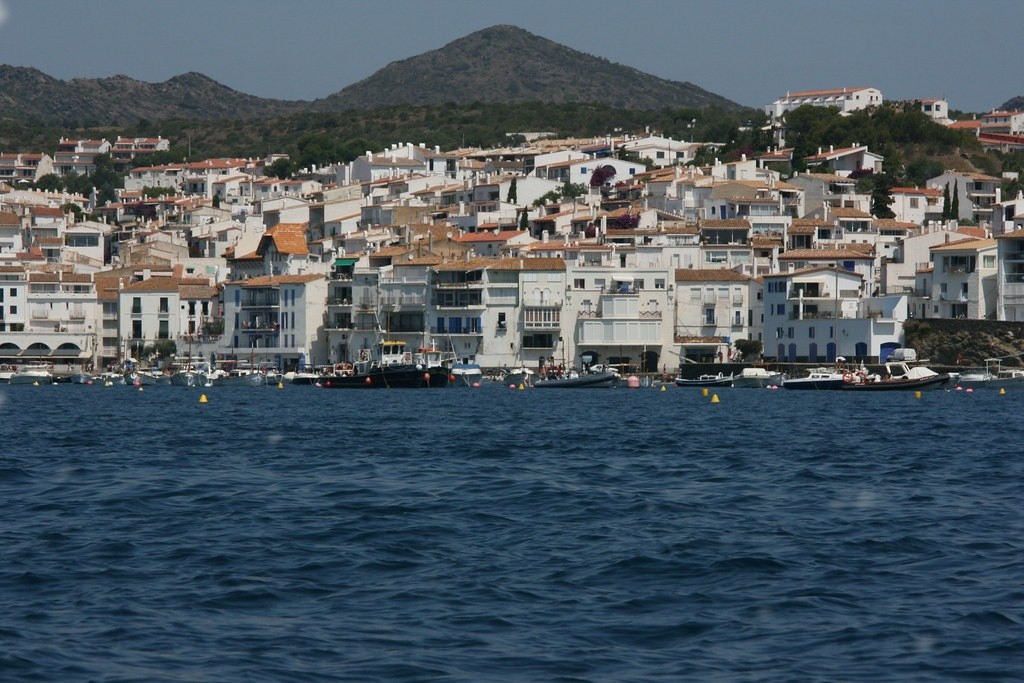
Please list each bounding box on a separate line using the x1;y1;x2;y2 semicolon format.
887;348;916;361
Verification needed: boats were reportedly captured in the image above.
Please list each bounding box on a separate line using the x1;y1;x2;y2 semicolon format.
675;357;1024;390
0;314;482;388
503;367;621;388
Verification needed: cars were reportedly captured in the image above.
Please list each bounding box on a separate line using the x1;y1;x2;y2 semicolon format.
589;364;618;374
